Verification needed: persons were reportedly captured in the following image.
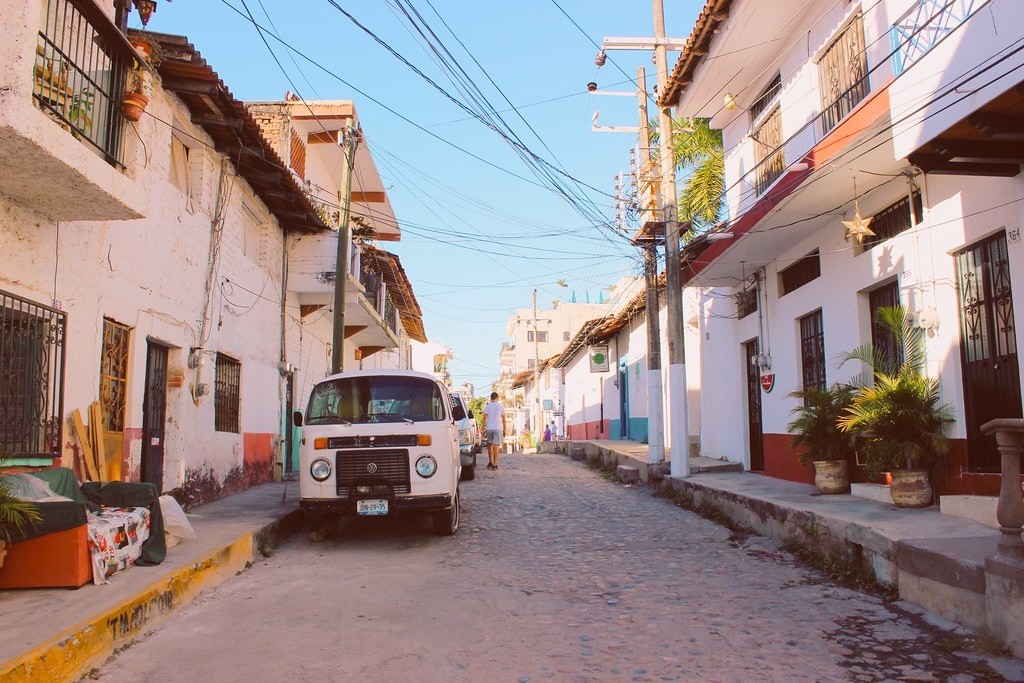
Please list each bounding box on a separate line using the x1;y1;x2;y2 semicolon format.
551;420;557;441
482;392;506;470
521;424;529;449
543;424;551;441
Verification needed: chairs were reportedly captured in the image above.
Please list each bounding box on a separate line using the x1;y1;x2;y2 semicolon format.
337;396;363;418
33;46;71;133
410;395;436;418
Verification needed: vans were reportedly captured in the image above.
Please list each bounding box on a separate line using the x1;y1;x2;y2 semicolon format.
292;369;464;535
383;392;477;481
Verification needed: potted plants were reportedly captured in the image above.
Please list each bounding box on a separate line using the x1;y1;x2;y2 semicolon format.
125;29;166;70
0;474;43;569
830;304;957;508
121;57;159;121
784;380;863;493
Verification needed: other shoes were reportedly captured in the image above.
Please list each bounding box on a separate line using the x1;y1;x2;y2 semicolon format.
492;465;498;471
487;463;494;469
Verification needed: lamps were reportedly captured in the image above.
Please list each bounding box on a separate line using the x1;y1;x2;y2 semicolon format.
725;93;753;115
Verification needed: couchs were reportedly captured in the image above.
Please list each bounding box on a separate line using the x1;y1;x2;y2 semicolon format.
0;467;159;588
